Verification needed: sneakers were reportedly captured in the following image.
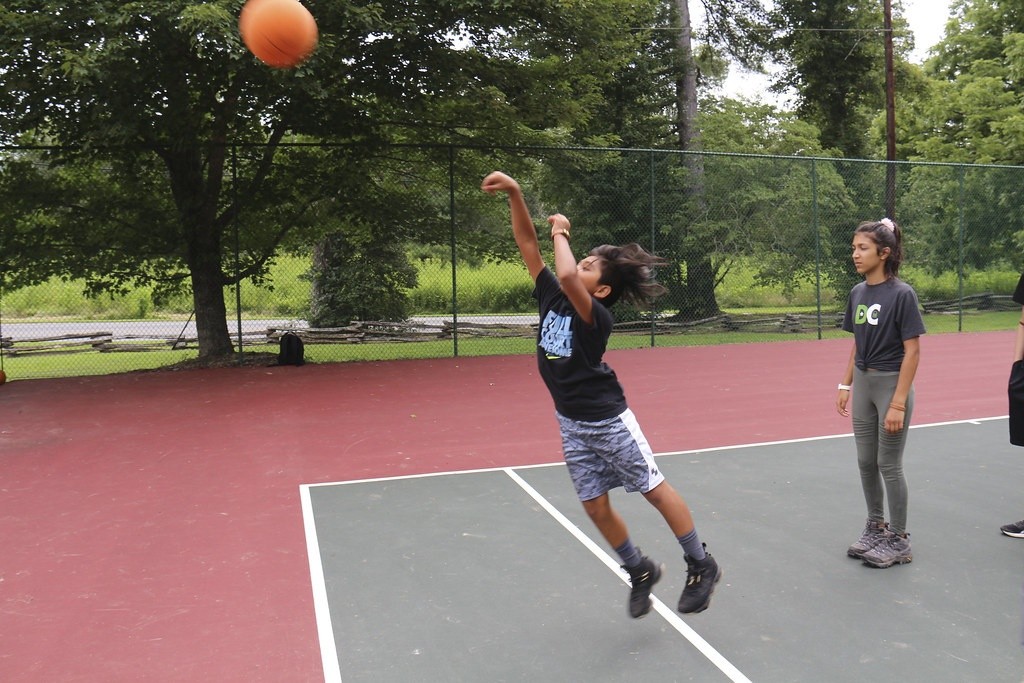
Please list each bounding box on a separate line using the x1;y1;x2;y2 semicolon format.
622;557;662;621
678;552;722;613
1001;520;1024;539
848;517;889;558
860;530;912;568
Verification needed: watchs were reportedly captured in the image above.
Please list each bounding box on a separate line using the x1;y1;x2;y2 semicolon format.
835;382;854;392
549;230;573;240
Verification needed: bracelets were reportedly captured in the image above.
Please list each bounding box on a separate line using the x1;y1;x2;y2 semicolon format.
889;402;908;413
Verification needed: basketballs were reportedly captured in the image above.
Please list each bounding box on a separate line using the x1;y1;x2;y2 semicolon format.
239;0;317;67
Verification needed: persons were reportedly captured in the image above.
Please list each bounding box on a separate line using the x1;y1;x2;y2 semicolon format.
831;216;926;569
482;171;721;620
1000;268;1024;542
